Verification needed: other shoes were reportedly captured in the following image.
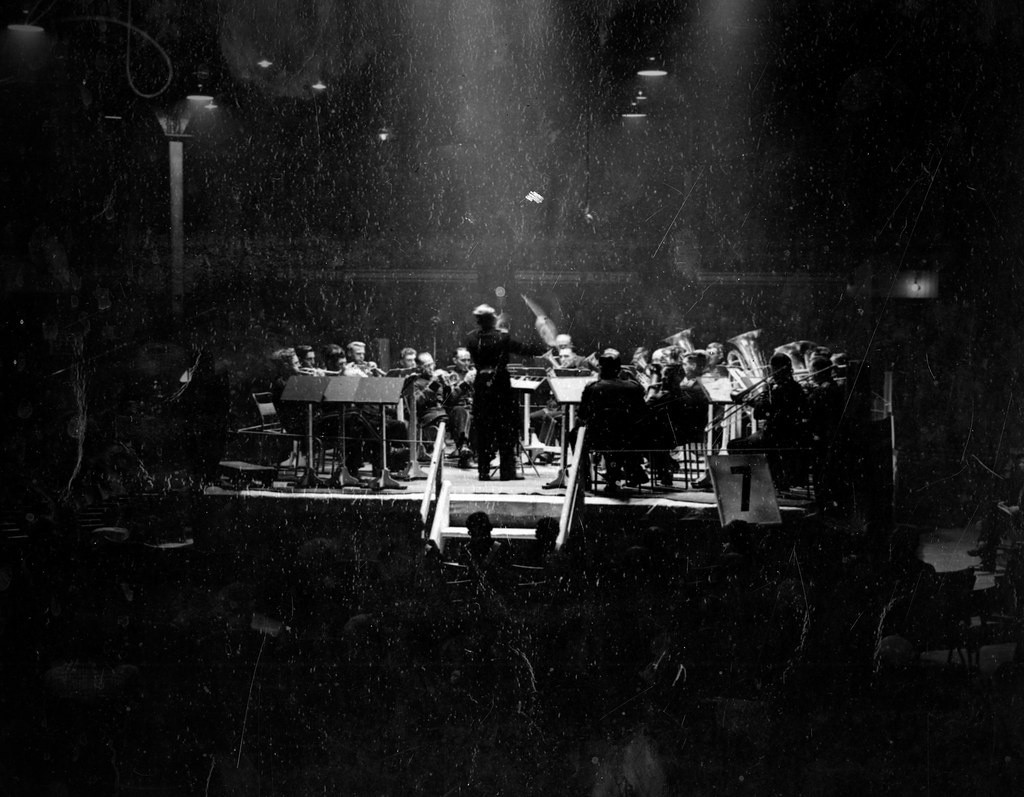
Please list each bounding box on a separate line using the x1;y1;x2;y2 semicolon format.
500;473;524;480
479;474;491;481
691;478;714;491
447;447;459;459
457;461;471;469
625;471;649;487
390;446;410;457
460;445;472;458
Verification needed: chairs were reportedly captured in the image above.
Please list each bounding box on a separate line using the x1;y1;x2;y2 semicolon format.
253;392;383;475
593;403;707;492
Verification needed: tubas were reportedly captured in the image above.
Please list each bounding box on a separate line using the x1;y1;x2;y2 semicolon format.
774;340;818;384
635;347;687;405
661;327;696;353
724;328;770;377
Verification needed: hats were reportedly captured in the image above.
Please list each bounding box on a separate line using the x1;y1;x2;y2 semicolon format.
472;303;495;318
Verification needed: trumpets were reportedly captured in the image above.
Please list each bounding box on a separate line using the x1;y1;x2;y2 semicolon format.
344;359;387;377
299;366;343;376
541;348;600;369
430;370;459;385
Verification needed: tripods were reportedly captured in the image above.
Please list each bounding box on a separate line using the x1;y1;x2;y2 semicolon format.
489;376;547;478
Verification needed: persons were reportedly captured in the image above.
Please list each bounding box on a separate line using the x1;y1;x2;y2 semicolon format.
464;303;545;482
0;296;1024;797
